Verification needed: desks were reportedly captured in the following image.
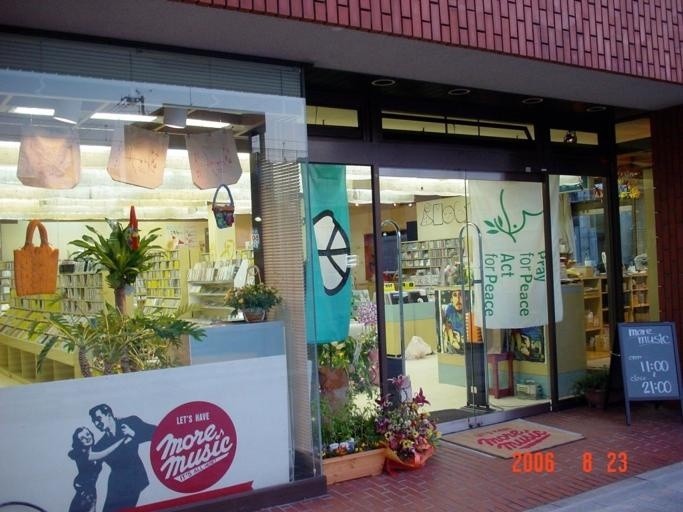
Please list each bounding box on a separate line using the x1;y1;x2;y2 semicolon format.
385;299;437;359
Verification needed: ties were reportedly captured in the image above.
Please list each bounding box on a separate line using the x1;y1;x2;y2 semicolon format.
107;427;115;440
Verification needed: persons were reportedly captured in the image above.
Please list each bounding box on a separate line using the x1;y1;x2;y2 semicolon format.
88;404;158;512
67;426;126;512
440;292;470;355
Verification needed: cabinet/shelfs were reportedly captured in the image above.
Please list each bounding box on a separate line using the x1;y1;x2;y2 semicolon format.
0;244;254;384
562;274;649;361
400;237;465;287
560;252;572;278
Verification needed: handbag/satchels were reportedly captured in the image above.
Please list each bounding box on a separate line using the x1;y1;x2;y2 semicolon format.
212;184;234;228
13;221;60;296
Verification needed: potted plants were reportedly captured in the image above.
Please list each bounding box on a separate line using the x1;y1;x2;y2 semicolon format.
568;364;616;409
310;388;386;485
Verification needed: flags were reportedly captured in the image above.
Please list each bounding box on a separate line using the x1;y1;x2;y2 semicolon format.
298;162;352;347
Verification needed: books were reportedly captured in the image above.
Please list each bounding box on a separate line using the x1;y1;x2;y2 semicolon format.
183;260;238;325
1;252;178;353
397;237;462;285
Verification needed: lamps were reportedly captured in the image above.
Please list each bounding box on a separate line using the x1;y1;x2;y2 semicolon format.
162;107;187;130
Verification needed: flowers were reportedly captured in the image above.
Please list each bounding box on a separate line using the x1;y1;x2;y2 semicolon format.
374;373;442;460
318;299;379;400
223;281;283;318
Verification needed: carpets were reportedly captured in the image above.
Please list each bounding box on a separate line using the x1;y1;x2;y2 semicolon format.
440;416;584;460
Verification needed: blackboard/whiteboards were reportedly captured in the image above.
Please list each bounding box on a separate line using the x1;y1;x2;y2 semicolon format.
620;323;679;400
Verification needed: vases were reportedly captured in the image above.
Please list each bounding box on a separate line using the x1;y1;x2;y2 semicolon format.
243;308;266;323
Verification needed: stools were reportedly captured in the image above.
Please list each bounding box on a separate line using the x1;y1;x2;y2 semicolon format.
487;352;514;399
